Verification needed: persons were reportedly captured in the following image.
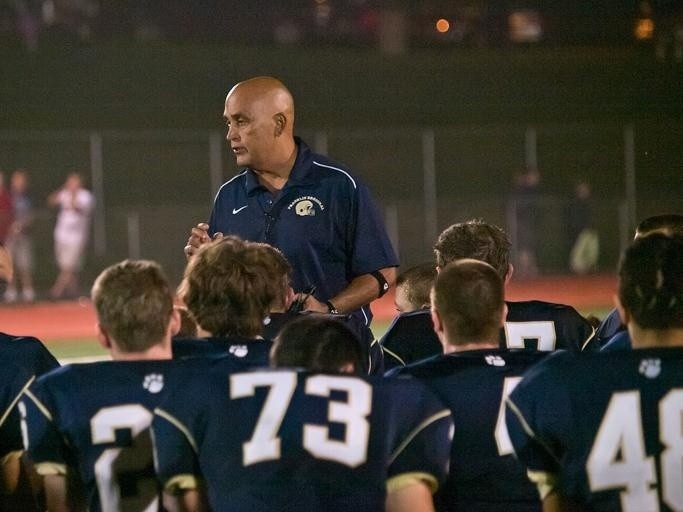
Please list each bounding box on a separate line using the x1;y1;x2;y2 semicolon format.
45;172;94;302
1;235;407;511
381;212;683;512
2;168;38;304
185;75;399;333
